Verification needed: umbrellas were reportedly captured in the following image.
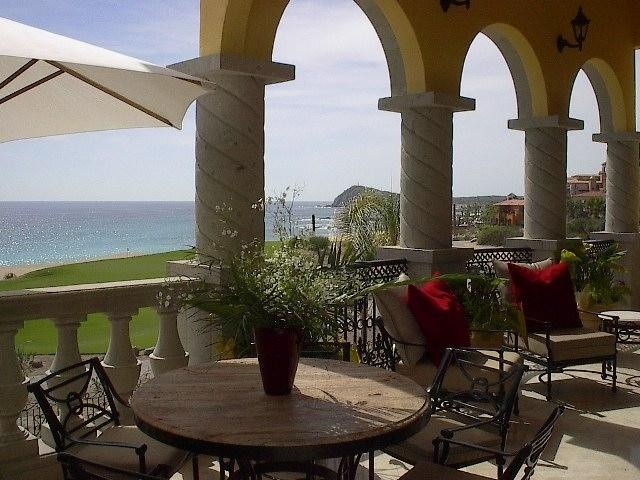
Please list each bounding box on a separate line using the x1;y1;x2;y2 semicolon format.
0;17;216;144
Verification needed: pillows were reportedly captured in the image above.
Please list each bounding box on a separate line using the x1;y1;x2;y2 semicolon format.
508;260;584;334
405;272;473;365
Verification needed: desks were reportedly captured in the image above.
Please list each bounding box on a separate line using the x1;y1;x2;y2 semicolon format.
128;356;434;480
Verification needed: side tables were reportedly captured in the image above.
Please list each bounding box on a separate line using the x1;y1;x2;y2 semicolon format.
596;309;640;380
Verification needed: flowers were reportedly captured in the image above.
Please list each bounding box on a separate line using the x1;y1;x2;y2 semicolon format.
156;179;374;343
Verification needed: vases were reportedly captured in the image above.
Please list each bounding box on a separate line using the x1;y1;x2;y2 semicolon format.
252;324;303;395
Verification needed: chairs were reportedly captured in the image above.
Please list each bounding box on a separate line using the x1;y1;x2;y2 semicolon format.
491;254;620;400
24;355;202;480
367;270;526;413
380;344;566;480
